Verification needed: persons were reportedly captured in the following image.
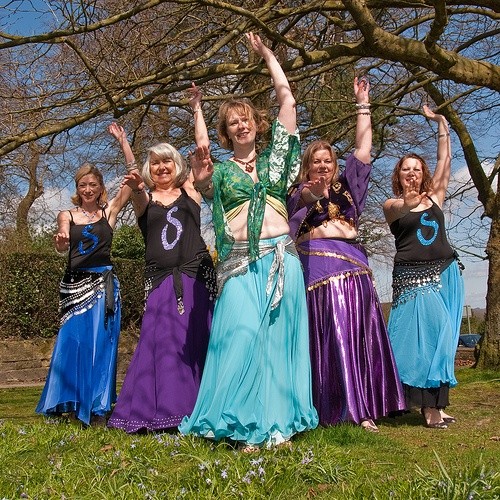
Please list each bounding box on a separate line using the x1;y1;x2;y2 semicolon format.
35;122;139;430
286;77;406;432
383;105;465;428
108;82;217;434
178;32;319;454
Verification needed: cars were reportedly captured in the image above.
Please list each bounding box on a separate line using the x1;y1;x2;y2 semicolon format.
457;333;482;348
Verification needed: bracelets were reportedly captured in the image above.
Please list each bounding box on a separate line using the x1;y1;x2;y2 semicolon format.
125;160;138;171
192;109;202;113
356;112;371;116
192;179;212;194
438;132;450;137
55;246;70;256
355;103;371;109
310;191;324;200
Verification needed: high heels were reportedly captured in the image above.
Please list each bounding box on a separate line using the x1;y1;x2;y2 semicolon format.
420;408;448;428
443;417;457;423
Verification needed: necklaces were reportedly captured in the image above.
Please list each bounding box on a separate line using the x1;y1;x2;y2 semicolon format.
233;154;257;172
80;205;99;222
